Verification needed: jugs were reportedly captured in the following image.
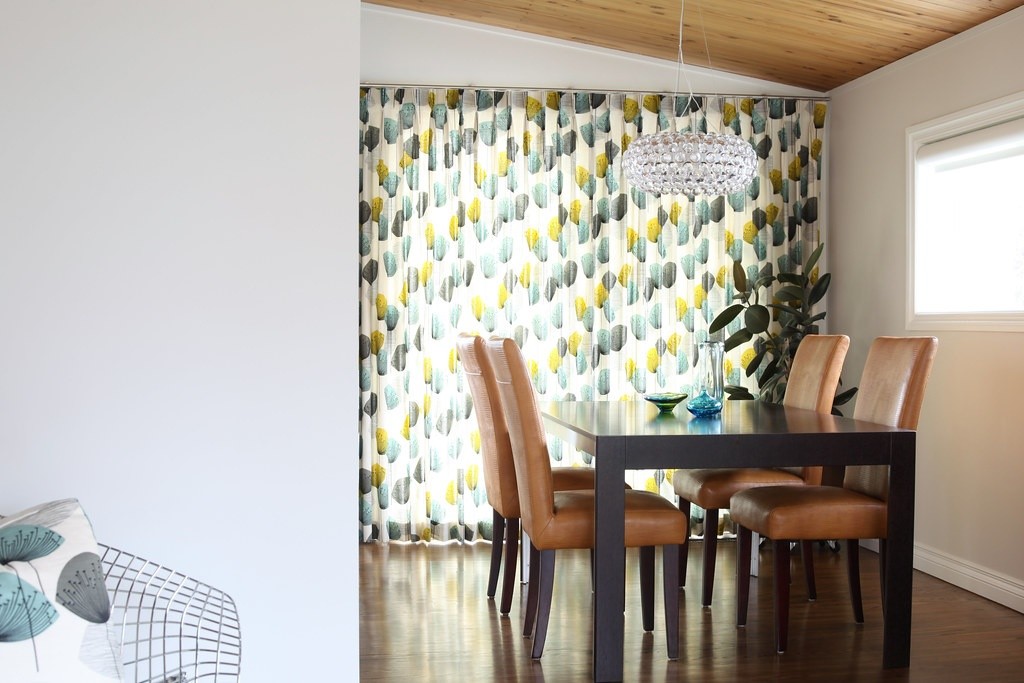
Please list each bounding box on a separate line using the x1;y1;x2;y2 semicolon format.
692;341;726;401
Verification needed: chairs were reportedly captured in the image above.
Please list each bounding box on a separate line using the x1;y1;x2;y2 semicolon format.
457;333;632;618
486;335;689;660
729;333;938;655
670;334;851;609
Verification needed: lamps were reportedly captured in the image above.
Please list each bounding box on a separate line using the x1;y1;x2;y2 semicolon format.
622;1;760;197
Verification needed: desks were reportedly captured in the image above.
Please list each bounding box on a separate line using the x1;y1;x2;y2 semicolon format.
542;396;920;682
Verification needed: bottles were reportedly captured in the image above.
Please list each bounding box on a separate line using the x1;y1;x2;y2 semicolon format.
686;389;722;418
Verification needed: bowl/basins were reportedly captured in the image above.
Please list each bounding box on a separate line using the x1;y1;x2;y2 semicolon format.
644;392;688;414
